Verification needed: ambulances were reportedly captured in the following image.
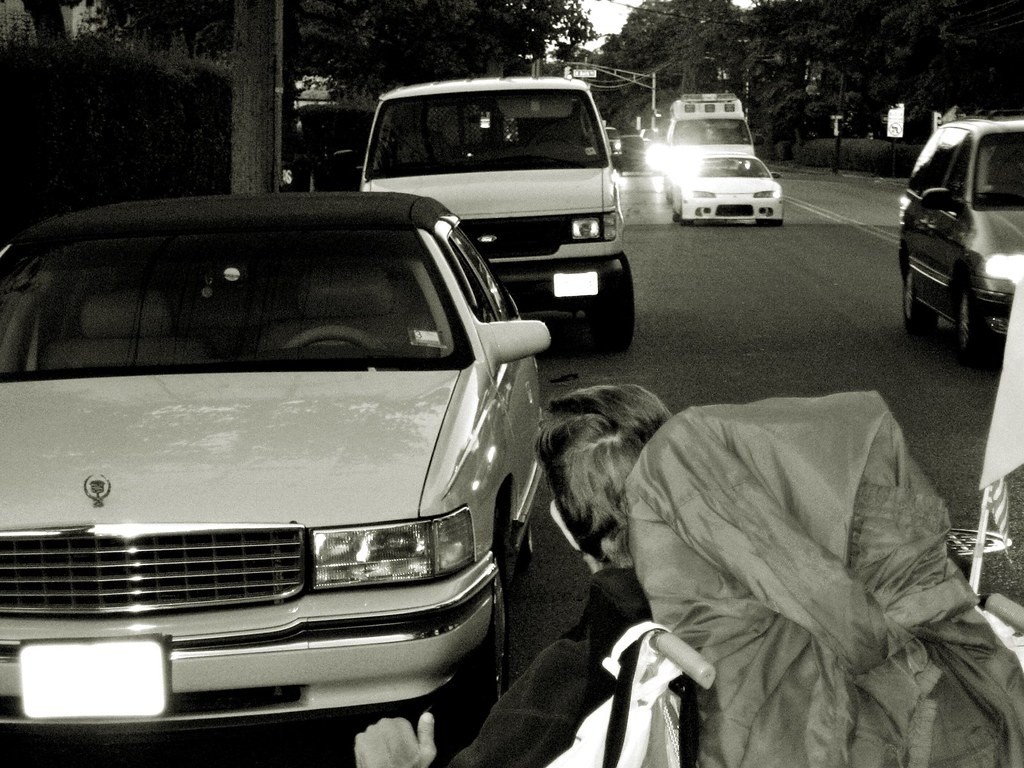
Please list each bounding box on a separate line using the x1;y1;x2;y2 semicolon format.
667;92;755;200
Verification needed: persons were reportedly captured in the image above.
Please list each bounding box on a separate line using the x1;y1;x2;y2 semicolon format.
355;382;678;768
540;386;1024;767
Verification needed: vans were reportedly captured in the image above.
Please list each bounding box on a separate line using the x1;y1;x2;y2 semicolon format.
360;74;635;354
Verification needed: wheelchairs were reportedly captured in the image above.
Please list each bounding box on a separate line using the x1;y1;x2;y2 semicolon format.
585;540;1024;768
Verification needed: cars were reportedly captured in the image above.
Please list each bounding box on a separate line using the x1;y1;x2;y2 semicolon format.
605;126;666;177
0;192;550;733
673;153;785;228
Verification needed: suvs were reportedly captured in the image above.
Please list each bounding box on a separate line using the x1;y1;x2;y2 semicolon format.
898;110;1024;369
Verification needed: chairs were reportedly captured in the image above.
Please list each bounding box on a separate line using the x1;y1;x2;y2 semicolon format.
42;289;216;371
257;260;436;355
